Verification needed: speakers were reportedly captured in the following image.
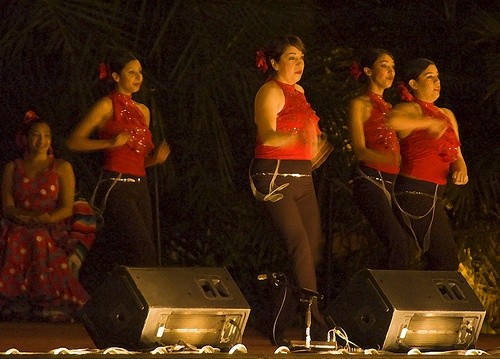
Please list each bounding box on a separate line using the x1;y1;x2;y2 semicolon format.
79;264;251;353
316;268;487;353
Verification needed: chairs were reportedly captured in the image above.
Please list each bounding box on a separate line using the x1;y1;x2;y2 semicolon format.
49;193;96;281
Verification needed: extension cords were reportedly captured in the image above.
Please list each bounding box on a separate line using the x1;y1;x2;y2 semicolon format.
290;340;337;351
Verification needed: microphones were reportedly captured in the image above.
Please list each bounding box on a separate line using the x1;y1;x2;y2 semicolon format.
257;272;284;280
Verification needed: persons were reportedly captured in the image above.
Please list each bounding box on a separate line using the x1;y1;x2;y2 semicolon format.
345;47;409;271
68;52;170;268
249;36;335;345
390;58;468;271
0;110;89;323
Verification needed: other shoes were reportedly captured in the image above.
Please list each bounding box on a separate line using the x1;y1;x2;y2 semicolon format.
270;336;292;346
